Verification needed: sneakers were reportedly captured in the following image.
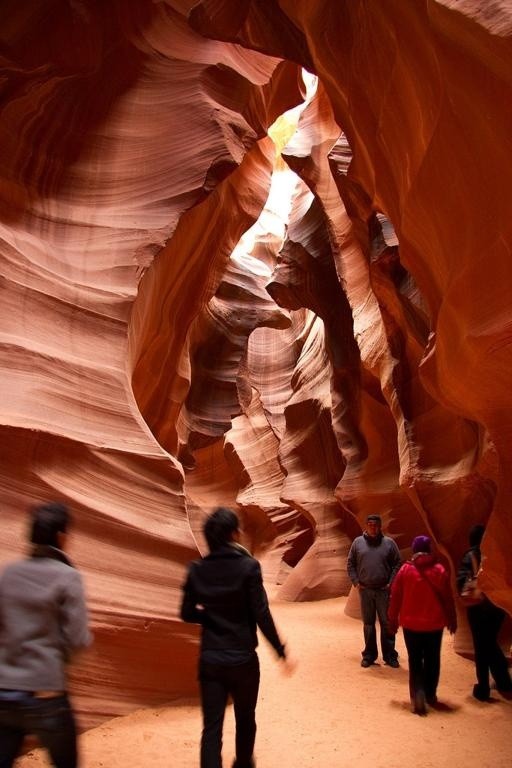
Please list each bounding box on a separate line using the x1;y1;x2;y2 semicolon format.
361;656;373;667
384;655;399;668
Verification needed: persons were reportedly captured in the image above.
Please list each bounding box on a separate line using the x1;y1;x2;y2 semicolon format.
457;524;512;701
347;515;403;668
387;536;457;717
179;507;297;768
0;503;95;768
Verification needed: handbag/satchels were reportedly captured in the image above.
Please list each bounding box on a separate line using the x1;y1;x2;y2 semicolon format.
344;584;362;621
458;574;485;607
